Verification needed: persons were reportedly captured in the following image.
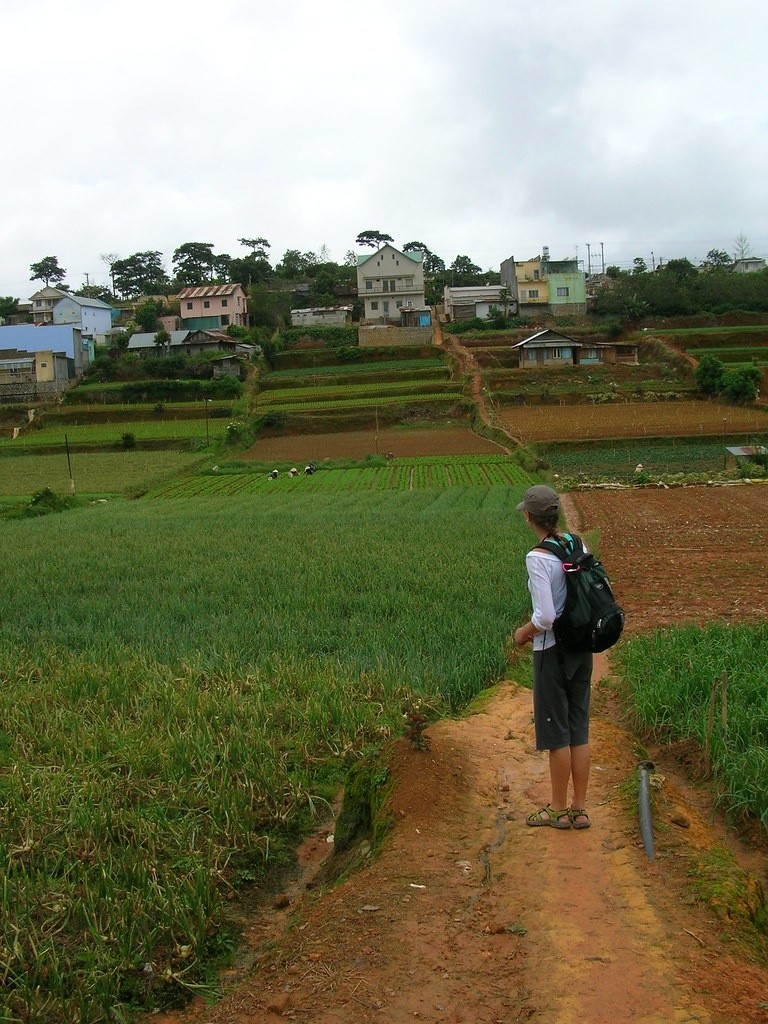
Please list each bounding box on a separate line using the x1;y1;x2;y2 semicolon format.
512;486;594;830
272;466;313;479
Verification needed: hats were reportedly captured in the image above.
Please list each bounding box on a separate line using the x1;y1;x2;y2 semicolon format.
273;469;278;472
291;468;297;472
515;484;560;516
306;466;310;469
637;463;643;467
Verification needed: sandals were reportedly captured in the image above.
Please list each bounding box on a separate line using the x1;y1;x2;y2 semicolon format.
566;806;590;828
526;803;571;828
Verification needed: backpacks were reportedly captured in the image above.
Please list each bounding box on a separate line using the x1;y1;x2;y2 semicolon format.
532;534;625;653
